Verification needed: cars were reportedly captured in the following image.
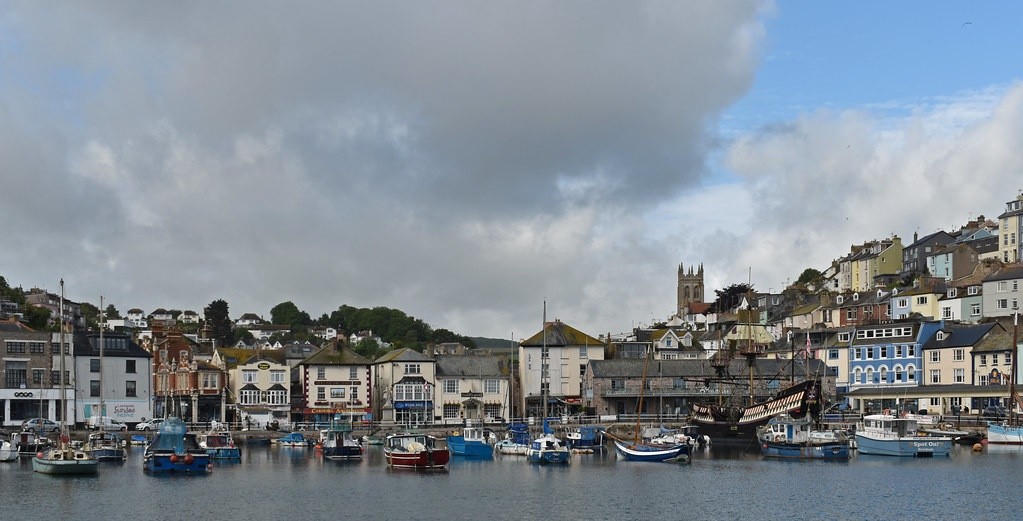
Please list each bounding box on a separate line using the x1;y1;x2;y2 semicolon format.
825;403;852;414
21;417;60;433
136;417;164;432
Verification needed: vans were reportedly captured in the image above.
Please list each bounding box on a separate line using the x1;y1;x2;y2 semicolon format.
89;415;128;433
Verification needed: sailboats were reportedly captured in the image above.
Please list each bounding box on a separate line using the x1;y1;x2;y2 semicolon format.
0;276;102;477
443;267;1023;465
80;296;132;463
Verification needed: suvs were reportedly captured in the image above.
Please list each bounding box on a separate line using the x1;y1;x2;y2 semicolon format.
982;405;1010;421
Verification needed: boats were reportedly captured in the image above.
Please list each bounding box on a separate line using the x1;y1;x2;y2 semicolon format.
131;341;386;476
383;429;451;469
